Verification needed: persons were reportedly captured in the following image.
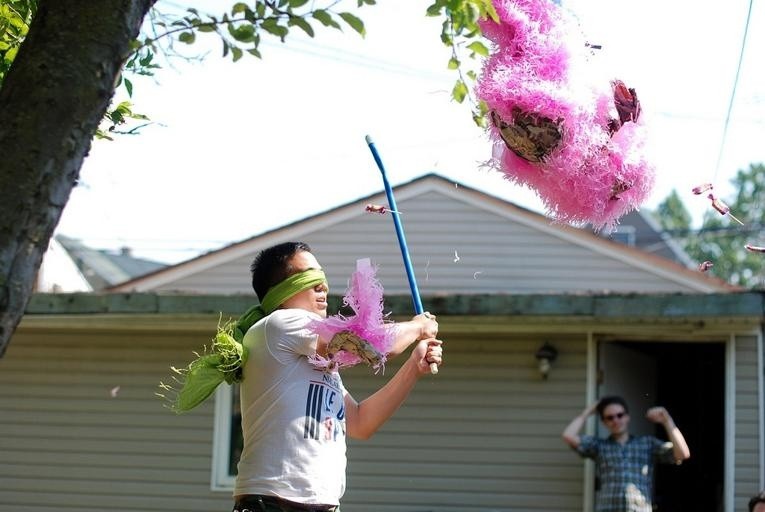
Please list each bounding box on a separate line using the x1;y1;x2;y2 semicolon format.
748;491;765;512
561;396;692;512
232;241;443;512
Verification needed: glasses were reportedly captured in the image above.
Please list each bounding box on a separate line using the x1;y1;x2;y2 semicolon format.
606;413;623;419
